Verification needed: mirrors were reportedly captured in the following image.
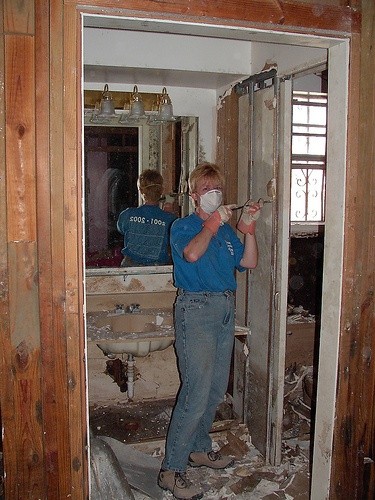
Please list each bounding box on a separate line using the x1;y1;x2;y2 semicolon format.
83;108;199;270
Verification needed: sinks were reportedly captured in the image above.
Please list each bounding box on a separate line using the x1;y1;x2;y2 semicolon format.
89;311;178;357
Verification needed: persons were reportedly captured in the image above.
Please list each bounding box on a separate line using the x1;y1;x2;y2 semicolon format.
117;170;178;267
157;162;263;500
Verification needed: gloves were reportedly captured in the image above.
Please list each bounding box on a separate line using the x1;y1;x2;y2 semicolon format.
202;204;239;236
236;198;264;235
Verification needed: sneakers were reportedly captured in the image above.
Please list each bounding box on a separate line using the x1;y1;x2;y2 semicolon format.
158;468;204;499
188;450;234;469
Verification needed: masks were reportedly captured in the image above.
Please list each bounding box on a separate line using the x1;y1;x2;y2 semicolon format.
195;189;223;215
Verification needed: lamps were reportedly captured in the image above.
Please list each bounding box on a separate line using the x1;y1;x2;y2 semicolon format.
119;97;136;125
154;86;176;122
89;100;105;123
146;94;164;126
99;84;120;119
123;85;148;120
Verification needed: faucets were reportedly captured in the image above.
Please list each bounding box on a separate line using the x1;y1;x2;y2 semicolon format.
126;306;133;313
